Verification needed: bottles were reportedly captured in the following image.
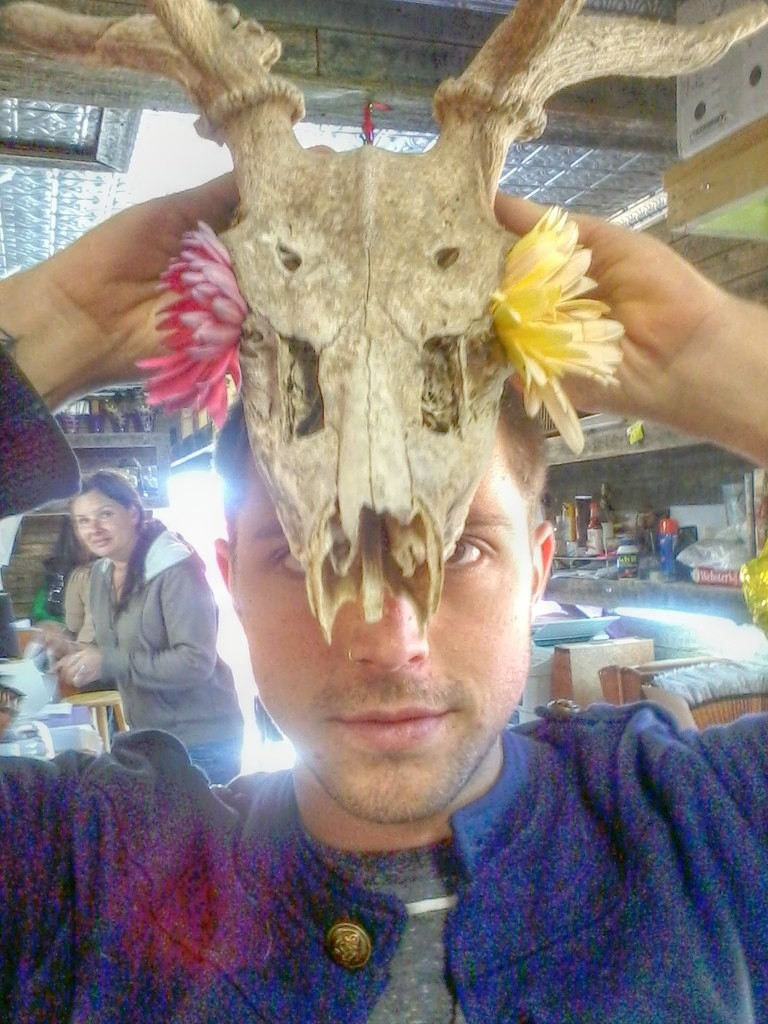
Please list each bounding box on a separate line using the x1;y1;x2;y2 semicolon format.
617;538;641;579
600;481;614;553
587;502;602;556
658;518;679;582
552;502;573;557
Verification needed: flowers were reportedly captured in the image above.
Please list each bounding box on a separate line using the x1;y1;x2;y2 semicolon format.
135;203;608;452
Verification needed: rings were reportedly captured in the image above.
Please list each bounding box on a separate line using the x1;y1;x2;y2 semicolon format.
81;664;86;672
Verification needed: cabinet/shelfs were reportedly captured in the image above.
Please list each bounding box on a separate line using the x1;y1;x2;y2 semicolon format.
53;384;170;510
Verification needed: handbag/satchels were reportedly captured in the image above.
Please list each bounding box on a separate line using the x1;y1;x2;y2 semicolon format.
43;517;82;619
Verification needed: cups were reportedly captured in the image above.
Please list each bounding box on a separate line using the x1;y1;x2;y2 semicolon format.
574;495;592;556
679;526;698;581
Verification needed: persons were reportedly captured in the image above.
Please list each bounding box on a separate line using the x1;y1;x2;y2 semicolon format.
0;143;768;1024
46;469;243;785
